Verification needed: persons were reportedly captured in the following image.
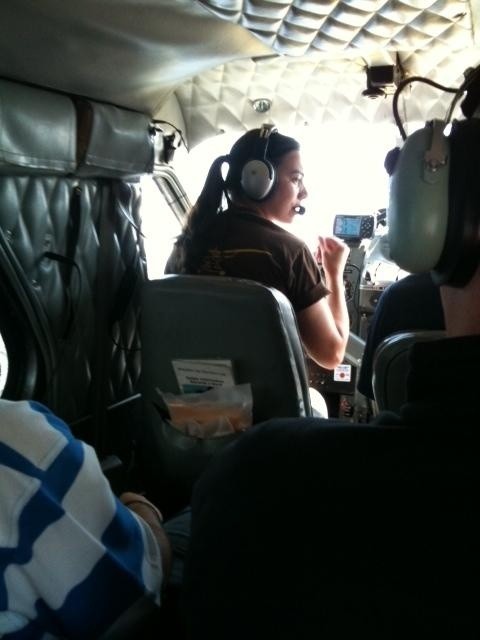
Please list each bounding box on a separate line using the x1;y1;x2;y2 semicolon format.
0;335;174;639
190;62;479;636
357;274;443;420
163;125;354;422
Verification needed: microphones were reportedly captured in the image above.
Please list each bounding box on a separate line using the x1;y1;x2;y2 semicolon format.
295;205;306;215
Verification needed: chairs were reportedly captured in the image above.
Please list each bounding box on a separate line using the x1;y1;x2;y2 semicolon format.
133;271;312;473
370;328;441;414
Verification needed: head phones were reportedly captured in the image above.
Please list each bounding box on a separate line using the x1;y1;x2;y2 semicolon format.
236;121;277;198
385;64;479;288
